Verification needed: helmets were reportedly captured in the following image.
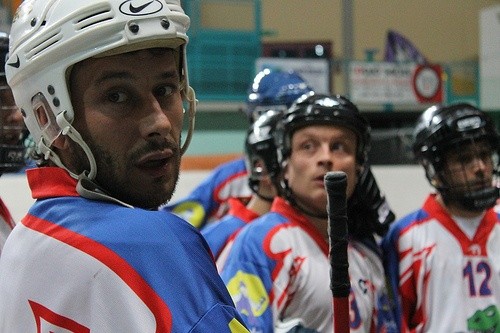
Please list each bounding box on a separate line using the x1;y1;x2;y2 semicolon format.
412;102;500;211
244;110;288;203
246;65;314;124
5;0;196;181
276;91;371;167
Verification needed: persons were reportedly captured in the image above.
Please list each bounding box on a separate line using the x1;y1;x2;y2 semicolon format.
0;0;250;333
380;100;500;333
163;69;397;333
